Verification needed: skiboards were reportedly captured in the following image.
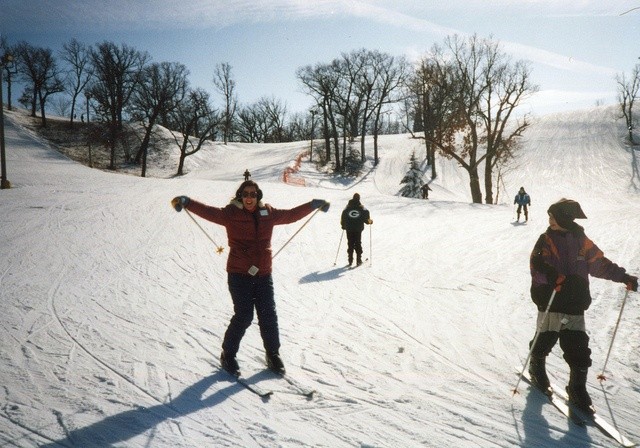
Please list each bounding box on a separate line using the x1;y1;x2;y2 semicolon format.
515;365;636;447
202;357;314;397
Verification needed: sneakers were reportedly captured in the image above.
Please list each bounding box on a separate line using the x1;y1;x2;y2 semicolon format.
565;379;592;408
265;353;285;374
220;352;241;376
529;364;553;394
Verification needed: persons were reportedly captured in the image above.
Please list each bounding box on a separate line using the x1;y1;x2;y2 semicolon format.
243;168;251;181
513;187;531;221
420;184;432;199
528;199;639;412
170;179;329;375
341;192;373;266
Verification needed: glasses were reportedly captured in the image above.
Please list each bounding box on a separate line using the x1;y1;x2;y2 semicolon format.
241;191;259;198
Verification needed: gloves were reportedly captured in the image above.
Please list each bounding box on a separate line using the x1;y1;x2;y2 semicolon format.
549;272;569;293
172;196;189;211
624;274;638;291
312;199;330;212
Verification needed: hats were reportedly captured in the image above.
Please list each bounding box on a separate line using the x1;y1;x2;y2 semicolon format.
548;197;587;229
